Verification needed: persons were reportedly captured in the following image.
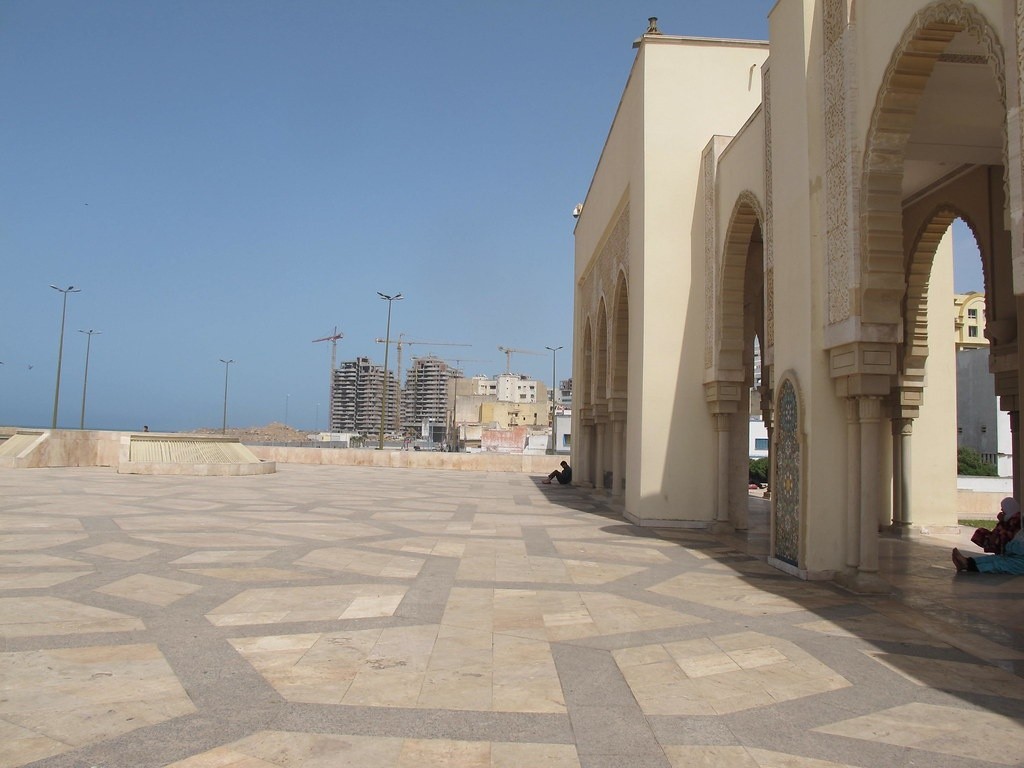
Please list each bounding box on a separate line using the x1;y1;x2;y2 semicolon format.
144;426;149;432
951;497;1024;574
542;461;572;484
749;480;767;489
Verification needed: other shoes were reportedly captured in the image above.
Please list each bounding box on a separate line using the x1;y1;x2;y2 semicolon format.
542;479;551;484
952;547;965;572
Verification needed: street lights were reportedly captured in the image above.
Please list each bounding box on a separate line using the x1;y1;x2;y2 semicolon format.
545;345;565;455
375;291;406;449
220;359;237;435
51;284;83;429
77;328;103;428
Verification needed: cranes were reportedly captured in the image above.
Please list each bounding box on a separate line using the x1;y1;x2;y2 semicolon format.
311;323;345;433
375;332;474;434
496;343;550;374
